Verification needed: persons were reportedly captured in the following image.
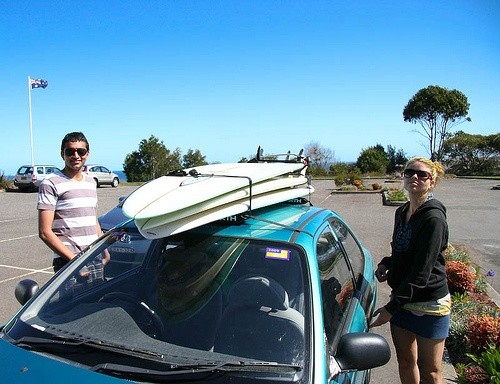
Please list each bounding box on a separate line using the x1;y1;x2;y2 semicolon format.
37;132;110;301
369;158;451;384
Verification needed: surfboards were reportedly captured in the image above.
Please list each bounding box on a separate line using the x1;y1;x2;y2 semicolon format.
122;158;315;241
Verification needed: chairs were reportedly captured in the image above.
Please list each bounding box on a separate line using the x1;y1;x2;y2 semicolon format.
136;243;304;363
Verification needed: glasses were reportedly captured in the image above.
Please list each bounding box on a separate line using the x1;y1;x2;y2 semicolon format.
64;148;88;156
403;169;433;181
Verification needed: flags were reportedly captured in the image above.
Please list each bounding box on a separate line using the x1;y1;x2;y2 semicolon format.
30;79;48;89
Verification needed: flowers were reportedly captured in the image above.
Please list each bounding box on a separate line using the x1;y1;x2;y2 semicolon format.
445;252;500;384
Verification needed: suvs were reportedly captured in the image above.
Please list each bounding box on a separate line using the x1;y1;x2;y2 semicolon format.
79;165;120;188
13;165;61;194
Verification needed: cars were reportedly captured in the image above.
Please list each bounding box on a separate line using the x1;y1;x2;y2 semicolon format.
0;194;391;384
